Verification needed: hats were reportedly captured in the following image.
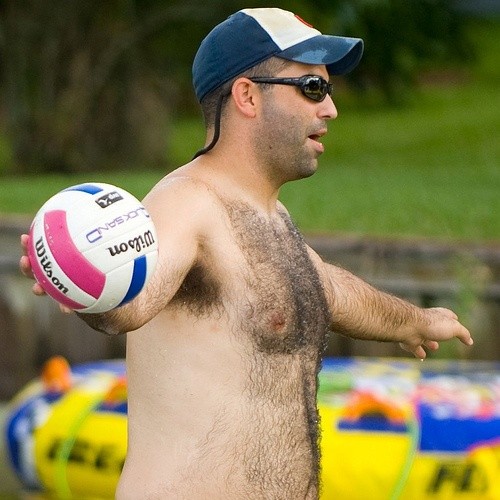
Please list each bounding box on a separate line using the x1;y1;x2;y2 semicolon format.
192;8;364;103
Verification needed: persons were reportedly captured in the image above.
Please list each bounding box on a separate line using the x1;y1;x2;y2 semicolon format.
17;5;475;499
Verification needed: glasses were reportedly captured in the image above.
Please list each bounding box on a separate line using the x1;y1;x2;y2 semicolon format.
226;74;333;102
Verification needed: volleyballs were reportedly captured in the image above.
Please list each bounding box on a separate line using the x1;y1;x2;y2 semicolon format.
25;182;158;315
